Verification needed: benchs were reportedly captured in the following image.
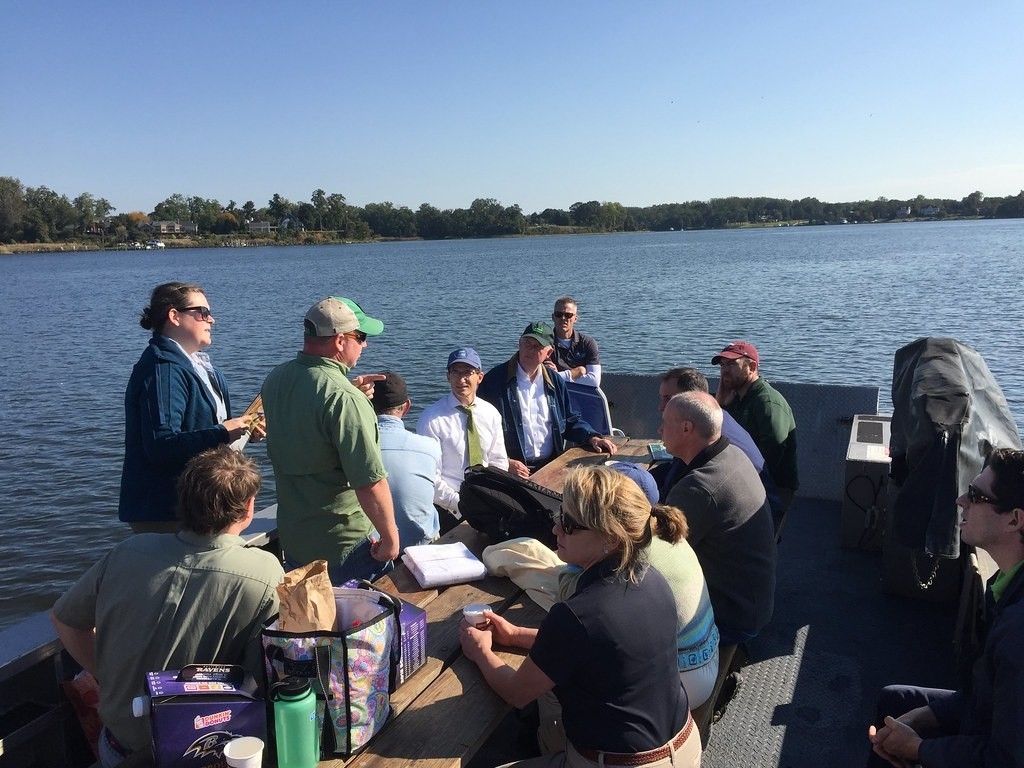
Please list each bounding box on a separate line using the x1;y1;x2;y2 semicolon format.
691;491;798;739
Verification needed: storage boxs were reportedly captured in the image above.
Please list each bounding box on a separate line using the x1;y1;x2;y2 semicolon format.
338;578;426;690
133;663;270;768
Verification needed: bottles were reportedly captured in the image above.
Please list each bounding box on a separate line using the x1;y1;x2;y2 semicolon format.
268;675;320;768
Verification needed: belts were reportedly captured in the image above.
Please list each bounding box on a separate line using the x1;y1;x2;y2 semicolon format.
571;712;710;767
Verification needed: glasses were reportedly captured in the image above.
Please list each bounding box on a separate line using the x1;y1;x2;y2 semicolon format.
556;505;596;536
448;370;476;378
343;332;369;342
181;304;212;320
964;482;1001;508
553;311;575;319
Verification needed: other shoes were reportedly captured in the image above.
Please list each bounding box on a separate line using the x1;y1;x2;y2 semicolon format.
712;672;743;725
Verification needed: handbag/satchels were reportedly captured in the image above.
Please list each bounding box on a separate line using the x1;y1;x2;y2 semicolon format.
256;584;396;761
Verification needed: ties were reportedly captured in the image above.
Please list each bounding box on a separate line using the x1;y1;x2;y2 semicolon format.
457;404;483;468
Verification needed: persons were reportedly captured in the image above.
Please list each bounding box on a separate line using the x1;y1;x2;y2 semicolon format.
543;296;601;387
459;460;720;768
869;447;1023;768
51;442;286;768
370;370;442;563
658;391;776;698
416;346;510;537
711;341;800;513
648;367;783;530
477;322;617;480
260;295;400;584
119;281;266;535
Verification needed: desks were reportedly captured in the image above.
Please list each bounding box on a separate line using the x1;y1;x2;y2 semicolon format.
316;435;673;768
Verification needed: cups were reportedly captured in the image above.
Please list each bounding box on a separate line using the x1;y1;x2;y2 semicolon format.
462;603;492;631
223;736;264;768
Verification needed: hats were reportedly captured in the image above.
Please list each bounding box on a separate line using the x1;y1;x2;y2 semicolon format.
371;369;409;408
302;295;385;336
444;347;482;371
521;321;555;348
711;341;761;364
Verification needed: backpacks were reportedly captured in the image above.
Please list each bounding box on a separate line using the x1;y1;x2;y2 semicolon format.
457;463;565;551
